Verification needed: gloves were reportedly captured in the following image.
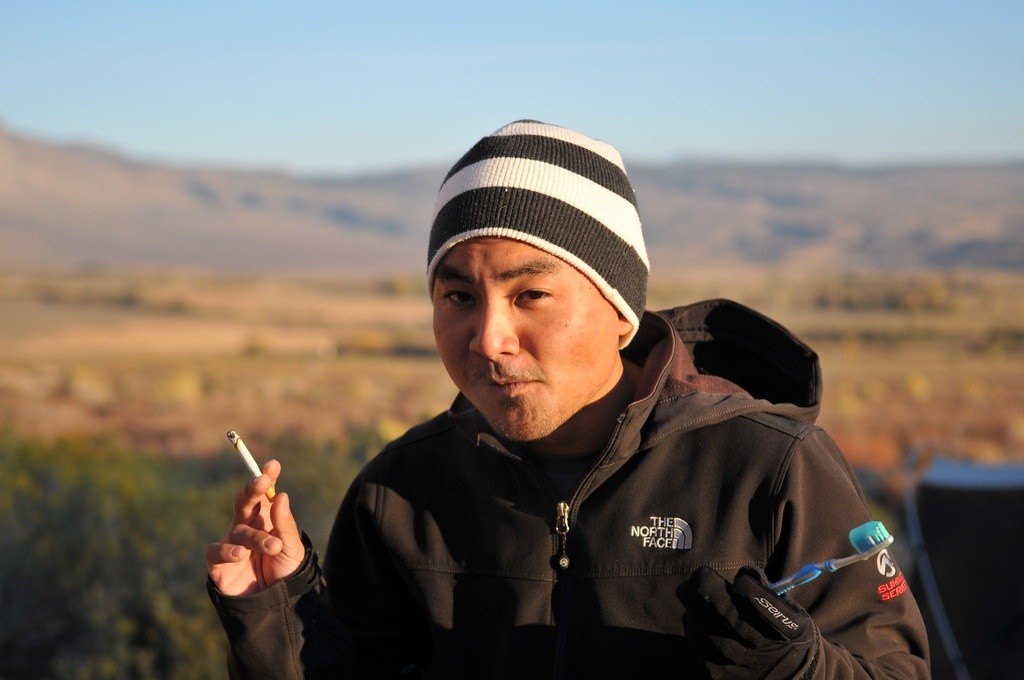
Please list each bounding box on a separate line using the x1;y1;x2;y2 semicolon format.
675;566;816;680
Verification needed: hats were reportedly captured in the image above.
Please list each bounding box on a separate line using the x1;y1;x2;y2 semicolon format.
426;119;650;351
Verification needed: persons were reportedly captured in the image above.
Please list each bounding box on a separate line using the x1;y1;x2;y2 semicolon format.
206;120;931;680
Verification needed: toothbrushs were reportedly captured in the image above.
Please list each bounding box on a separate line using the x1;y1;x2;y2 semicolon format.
768;519;894;597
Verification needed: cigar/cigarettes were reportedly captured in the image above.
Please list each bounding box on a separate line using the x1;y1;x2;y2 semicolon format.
227;430;276;503
